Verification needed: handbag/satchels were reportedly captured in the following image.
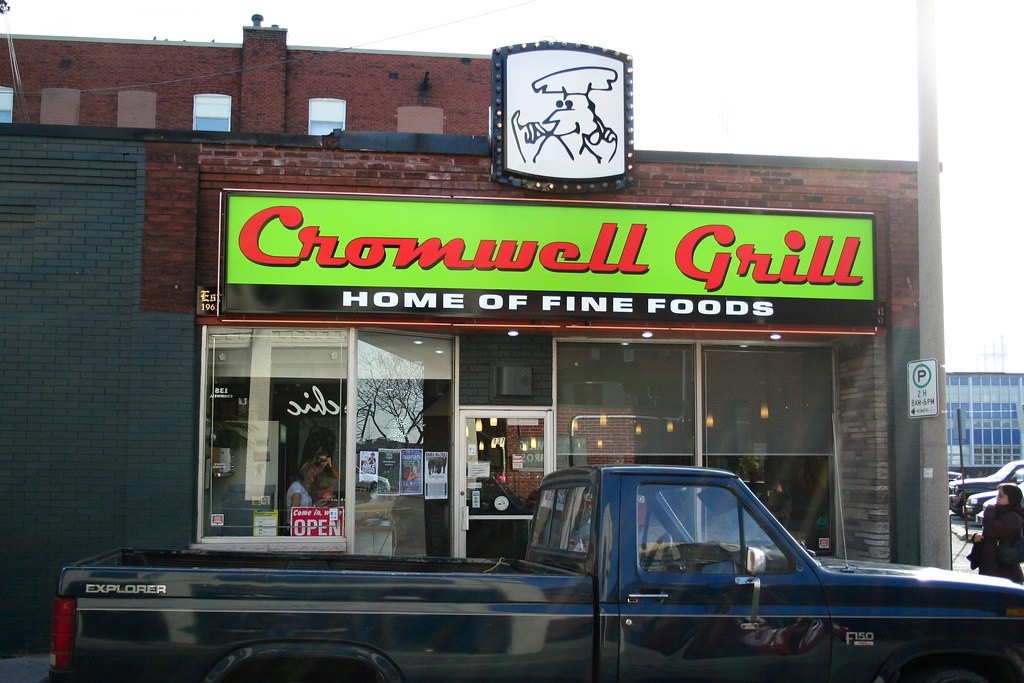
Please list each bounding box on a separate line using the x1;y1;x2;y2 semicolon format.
966;533;981;569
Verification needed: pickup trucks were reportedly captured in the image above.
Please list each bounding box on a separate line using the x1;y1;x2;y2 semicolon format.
46;461;1024;683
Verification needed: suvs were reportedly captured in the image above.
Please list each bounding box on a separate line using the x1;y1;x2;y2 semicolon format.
356;466;391;498
949;461;1024;516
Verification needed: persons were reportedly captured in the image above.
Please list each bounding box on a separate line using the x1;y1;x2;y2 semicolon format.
285;450;338;535
966;483;1024;584
428;453;447;475
949;494;959;510
368;453;376;464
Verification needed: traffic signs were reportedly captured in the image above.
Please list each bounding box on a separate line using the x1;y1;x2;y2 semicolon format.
906;358;939;420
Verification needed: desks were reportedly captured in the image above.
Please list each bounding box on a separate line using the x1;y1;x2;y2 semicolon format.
355;503;413;556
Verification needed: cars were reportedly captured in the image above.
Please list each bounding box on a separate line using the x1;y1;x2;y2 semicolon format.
963;481;1024;528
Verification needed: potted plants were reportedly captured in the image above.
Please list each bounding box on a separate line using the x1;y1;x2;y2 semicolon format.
738;453;762;482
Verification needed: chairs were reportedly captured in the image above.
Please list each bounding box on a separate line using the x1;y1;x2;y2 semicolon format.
765;481;794;530
